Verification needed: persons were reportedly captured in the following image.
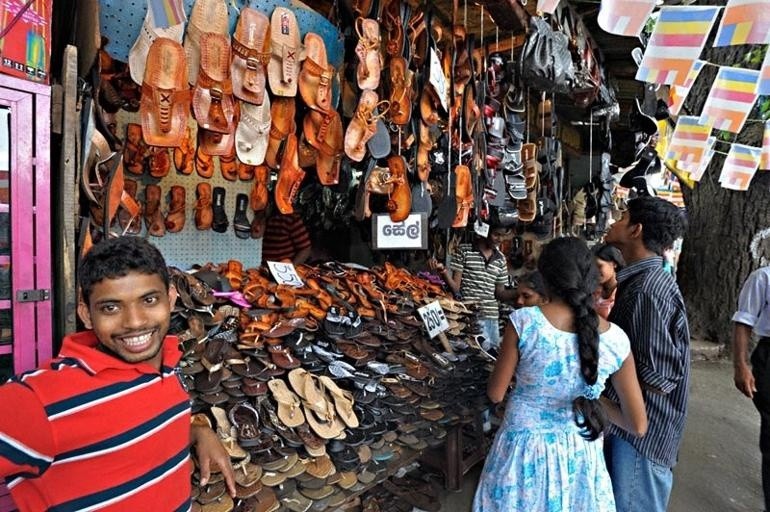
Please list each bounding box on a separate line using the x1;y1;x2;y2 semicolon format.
429;198;690;512
0;236;238;512
262;203;312;267
731;266;770;512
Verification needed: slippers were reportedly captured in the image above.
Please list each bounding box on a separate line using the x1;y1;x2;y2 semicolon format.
79;0;273;265
345;17;567;270
169;259;520;512
624;43;670;200
298;30;334;115
298;107;335;169
265;6;302;98
571;151;618;242
316;111;342;191
263;96;298;171
272;133;306;217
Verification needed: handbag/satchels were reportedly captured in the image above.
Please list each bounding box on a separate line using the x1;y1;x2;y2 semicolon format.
514;0;621;126
